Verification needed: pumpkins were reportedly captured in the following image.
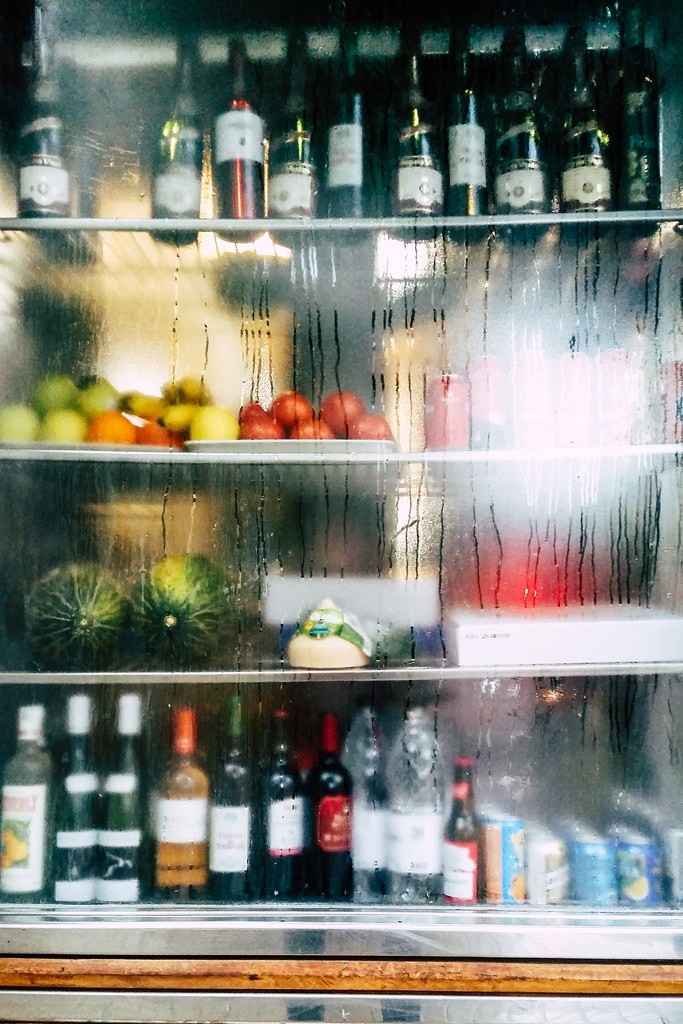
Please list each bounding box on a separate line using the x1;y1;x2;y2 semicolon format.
24;555;226;664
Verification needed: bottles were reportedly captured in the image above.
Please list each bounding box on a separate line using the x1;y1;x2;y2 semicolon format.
52;693;101;902
259;701;311;904
354;709;387;905
0;704;51;902
387;708;443;904
491;7;551;241
321;27;378;242
554;23;619;237
618;4;660;238
443;756;480;902
150;33;204;245
310;712;353;905
15;39;100;262
266;26;320;244
210;29;265;239
153;710;206;900
102;694;153;903
444;27;491;240
387;24;443;239
206;694;258;900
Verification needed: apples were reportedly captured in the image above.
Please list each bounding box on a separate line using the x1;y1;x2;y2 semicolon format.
0;375;121;442
190;392;386;441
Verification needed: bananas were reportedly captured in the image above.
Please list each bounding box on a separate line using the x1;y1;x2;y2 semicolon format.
121;375;212;430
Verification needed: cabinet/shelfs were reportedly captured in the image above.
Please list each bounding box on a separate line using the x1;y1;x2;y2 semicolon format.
0;208;683;964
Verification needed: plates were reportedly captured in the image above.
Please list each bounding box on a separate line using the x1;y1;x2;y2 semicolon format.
0;440;184;452
184;437;396;457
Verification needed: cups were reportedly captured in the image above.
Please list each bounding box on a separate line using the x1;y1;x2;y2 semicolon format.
422;374;466;451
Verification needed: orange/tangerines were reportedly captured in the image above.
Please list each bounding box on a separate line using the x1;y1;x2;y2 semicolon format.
86;414;186;448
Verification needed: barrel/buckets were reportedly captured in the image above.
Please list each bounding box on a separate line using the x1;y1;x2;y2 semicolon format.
77;496;222;582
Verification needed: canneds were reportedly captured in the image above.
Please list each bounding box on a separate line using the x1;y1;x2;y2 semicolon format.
422;372;473;452
473;790;683;905
